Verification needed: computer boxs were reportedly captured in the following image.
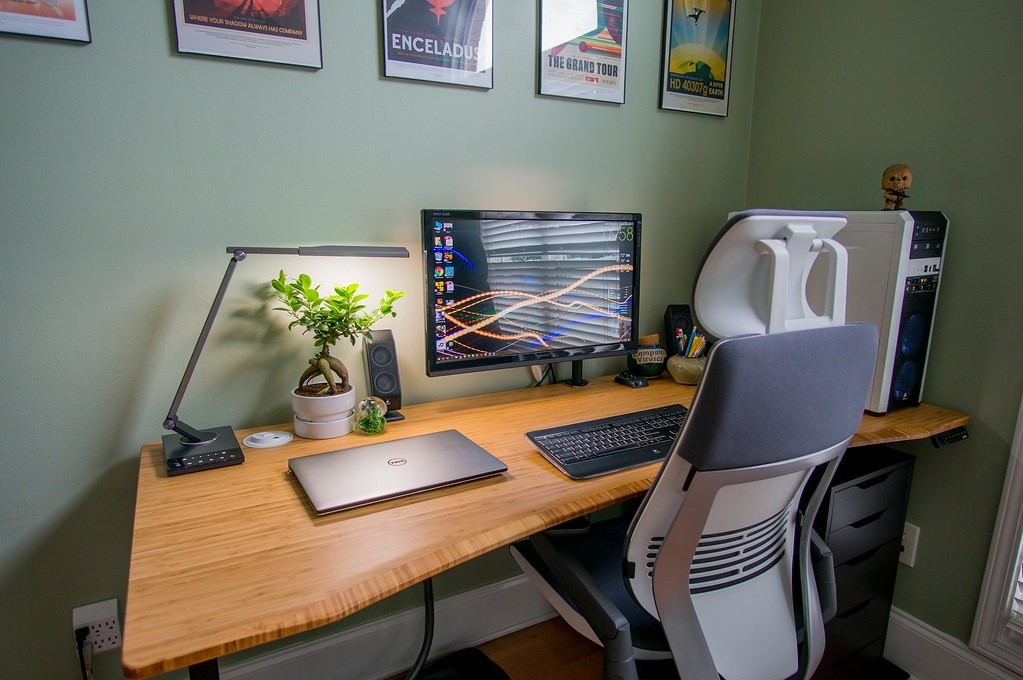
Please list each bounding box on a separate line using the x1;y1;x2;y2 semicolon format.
805;209;950;415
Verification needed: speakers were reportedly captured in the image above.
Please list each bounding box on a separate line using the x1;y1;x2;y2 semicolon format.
362;330;405;422
664;304;693;356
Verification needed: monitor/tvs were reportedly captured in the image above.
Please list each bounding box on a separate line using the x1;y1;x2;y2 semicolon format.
419;209;642;384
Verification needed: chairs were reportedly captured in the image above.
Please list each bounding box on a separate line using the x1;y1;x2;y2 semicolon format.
510;211;880;680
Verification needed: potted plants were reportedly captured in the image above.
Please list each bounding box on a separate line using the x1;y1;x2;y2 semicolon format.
270;269;408;439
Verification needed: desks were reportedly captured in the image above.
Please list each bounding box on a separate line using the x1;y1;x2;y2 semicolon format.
122;369;974;680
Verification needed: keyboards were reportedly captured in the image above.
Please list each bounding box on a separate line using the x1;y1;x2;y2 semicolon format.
525;403;689;479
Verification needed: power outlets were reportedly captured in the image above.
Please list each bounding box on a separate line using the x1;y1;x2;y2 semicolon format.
72;598;122;656
899;521;921;568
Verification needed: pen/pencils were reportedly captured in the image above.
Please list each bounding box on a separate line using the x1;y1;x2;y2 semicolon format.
676;325;707;358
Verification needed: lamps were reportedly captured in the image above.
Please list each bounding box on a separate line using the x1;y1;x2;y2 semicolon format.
161;245;411;477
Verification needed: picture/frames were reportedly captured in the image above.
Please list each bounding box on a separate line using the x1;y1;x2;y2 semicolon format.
0;0;92;44
538;0;629;105
172;0;323;70
382;0;494;90
658;0;737;117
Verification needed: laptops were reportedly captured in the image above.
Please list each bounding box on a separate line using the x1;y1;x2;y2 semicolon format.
288;429;509;515
